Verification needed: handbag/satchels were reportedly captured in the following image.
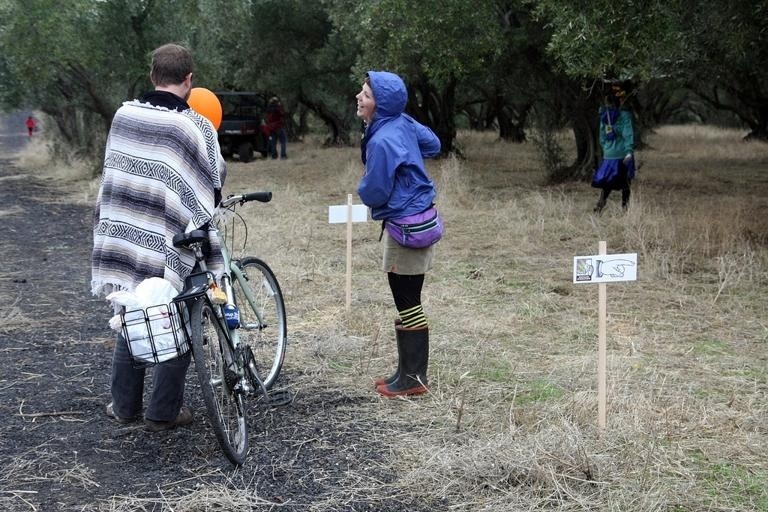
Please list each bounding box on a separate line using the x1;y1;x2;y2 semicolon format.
379;204;443;248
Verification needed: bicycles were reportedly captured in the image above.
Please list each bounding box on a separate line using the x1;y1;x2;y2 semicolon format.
172;192;288;465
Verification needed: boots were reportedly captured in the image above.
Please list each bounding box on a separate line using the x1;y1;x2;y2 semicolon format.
375;319;428;396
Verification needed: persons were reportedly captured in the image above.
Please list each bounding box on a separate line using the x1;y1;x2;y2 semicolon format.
90;44;228;432
591;89;635;217
264;97;289;160
26;114;35;139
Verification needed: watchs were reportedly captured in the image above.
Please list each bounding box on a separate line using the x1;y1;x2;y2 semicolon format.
355;70;445;396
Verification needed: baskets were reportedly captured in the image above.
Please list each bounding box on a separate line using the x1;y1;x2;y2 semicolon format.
121;301;193;369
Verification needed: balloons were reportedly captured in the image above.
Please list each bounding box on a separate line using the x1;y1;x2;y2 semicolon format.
185;87;224;131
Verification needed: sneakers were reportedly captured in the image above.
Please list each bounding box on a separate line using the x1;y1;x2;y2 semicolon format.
106;402;193;431
